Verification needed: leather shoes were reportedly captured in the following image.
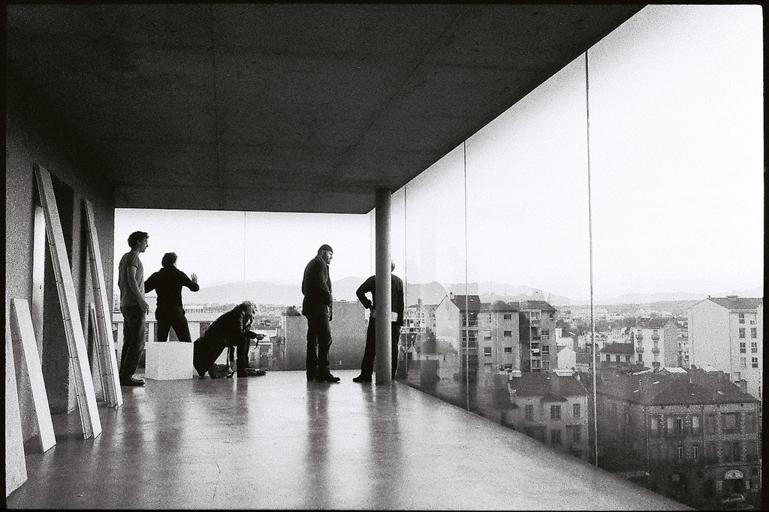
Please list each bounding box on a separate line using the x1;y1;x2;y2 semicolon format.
353;374;371;382
307;373;340;382
120;377;145;386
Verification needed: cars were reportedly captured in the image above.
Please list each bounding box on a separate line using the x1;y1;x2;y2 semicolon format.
721;492;746;506
726;503;755;510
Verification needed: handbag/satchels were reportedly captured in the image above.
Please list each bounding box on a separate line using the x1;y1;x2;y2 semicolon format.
208;363;230;379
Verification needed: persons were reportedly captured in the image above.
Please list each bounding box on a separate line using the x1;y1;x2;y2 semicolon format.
118;231;151;386
302;244;340;383
353;261;405;383
145;252;199;343
203;301;266;376
